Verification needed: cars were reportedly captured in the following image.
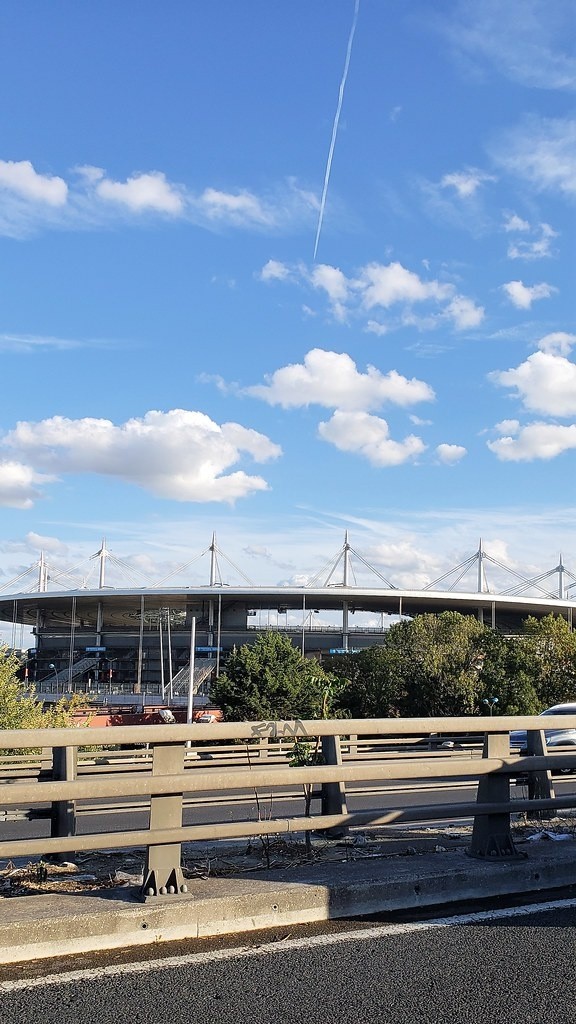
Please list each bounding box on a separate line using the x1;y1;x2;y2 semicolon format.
509;701;576;776
198;714;217;724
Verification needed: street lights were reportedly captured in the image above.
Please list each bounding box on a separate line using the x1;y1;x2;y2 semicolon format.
49;664;58;715
